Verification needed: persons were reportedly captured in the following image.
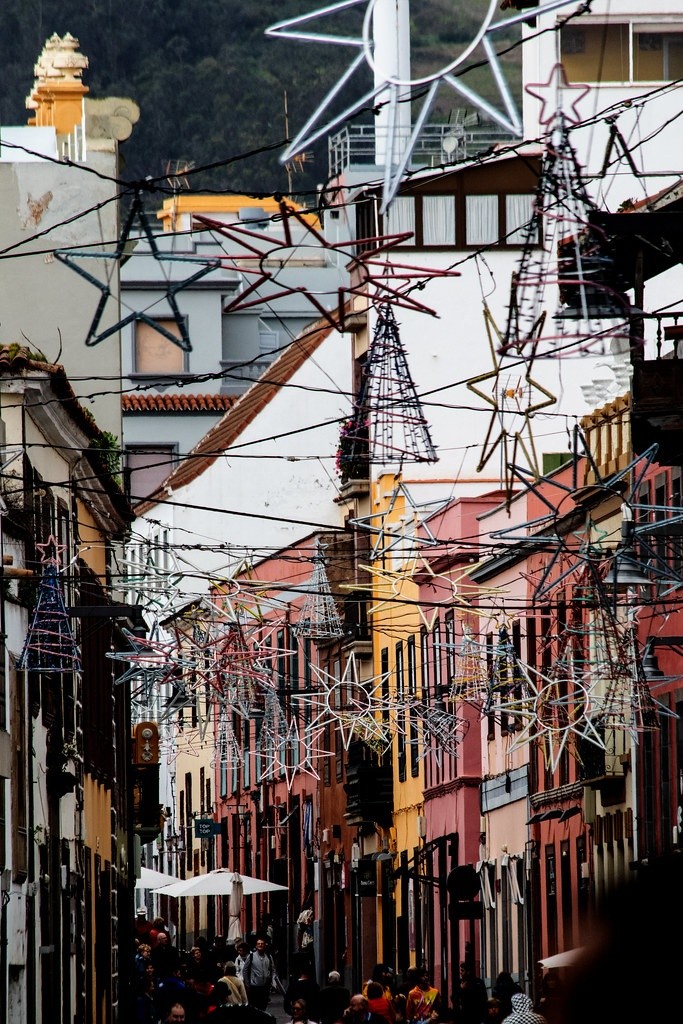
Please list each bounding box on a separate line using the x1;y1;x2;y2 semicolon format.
131;909;547;1024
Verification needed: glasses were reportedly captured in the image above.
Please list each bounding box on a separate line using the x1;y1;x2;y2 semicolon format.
292;1006;302;1013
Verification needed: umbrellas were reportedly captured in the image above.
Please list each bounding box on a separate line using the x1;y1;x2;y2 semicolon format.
135;867;288;939
